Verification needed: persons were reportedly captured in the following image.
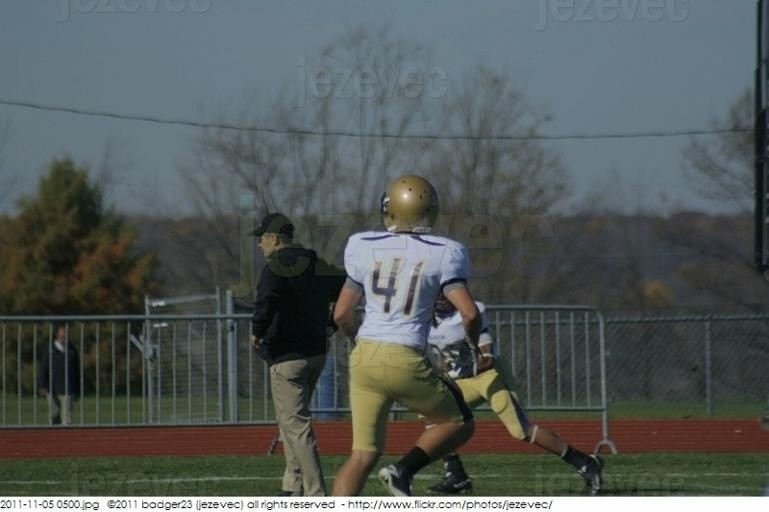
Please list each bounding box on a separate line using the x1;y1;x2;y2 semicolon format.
424;287;604;497
35;321;79;425
246;212;350;497
329;174;482;497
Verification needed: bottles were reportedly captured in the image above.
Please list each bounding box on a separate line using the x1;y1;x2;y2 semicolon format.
246;211;295;238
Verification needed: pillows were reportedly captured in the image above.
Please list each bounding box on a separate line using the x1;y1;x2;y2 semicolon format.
379;173;441;235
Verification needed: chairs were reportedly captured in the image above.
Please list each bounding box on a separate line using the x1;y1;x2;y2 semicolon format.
574;453;607;496
425;473;474;496
376;462;415;496
